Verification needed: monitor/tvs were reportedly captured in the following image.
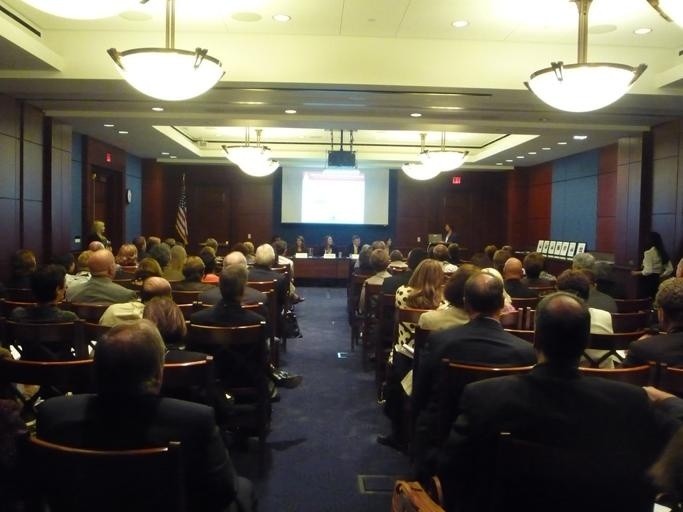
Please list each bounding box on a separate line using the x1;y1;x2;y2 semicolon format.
428;234;442;243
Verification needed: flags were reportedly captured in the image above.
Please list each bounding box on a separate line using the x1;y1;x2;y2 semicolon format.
176;176;190;245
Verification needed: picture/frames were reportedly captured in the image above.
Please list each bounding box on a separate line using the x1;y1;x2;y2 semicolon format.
536;239;587;259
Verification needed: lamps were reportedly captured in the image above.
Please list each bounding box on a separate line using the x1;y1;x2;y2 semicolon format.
20;0;148;21
402;134;441;180
646;0;683;29
221;128;271;166
419;131;470;172
524;0;648;112
106;0;226;101
238;130;280;178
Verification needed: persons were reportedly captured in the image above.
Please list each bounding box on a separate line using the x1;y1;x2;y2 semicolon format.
291;236;309;255
2;220;305;512
346;236;362;257
355;224;683;512
320;236;338;256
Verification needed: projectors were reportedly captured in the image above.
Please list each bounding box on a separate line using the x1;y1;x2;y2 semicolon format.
328;150;355;167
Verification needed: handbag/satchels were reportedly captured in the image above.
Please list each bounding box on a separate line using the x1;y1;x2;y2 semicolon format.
284;315;302;338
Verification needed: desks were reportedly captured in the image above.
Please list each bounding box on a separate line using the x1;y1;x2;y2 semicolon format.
290;256;337;286
337;258;352;286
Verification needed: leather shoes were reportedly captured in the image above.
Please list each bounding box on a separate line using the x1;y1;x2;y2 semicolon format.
272;368;303;389
270;392;280;402
291;296;305;305
